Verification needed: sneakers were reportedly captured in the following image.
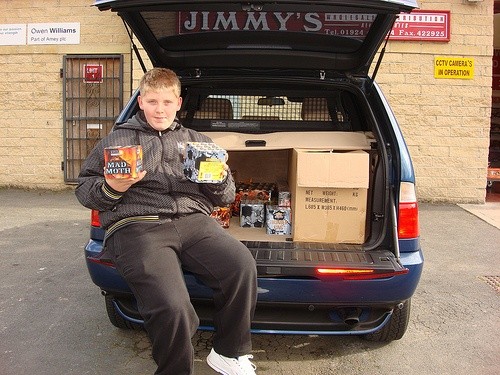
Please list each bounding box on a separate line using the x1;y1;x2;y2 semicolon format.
206;347;257;375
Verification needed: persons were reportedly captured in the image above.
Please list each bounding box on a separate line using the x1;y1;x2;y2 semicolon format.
76;65;259;375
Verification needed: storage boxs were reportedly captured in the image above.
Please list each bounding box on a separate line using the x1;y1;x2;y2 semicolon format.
182;141;230;184
232;181;277;217
104;145;143;180
276;178;290;207
208;204;231;228
287;147;369;244
266;203;292;236
239;198;265;229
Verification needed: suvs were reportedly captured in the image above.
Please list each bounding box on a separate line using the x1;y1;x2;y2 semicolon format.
80;0;427;343
